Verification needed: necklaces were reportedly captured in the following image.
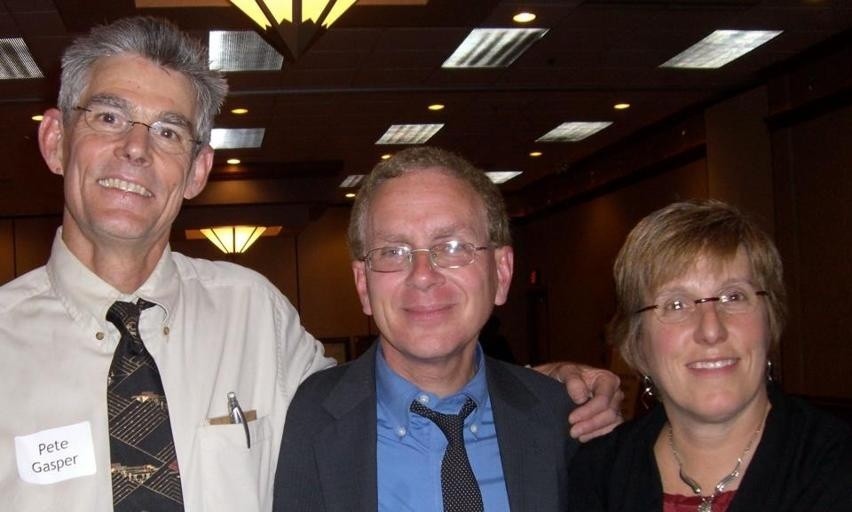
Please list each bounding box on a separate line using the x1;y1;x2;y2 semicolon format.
662;416;766;512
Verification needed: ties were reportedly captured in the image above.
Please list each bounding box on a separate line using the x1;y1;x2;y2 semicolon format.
105;298;186;512
410;396;486;512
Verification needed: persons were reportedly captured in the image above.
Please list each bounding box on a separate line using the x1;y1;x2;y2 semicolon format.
264;144;594;512
0;11;630;511
558;191;852;512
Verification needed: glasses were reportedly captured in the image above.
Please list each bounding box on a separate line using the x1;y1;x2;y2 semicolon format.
635;285;771;324
72;105;202;154
358;241;495;273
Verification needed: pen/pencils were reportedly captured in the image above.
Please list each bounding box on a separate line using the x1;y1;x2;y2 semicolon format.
227;392;250;448
